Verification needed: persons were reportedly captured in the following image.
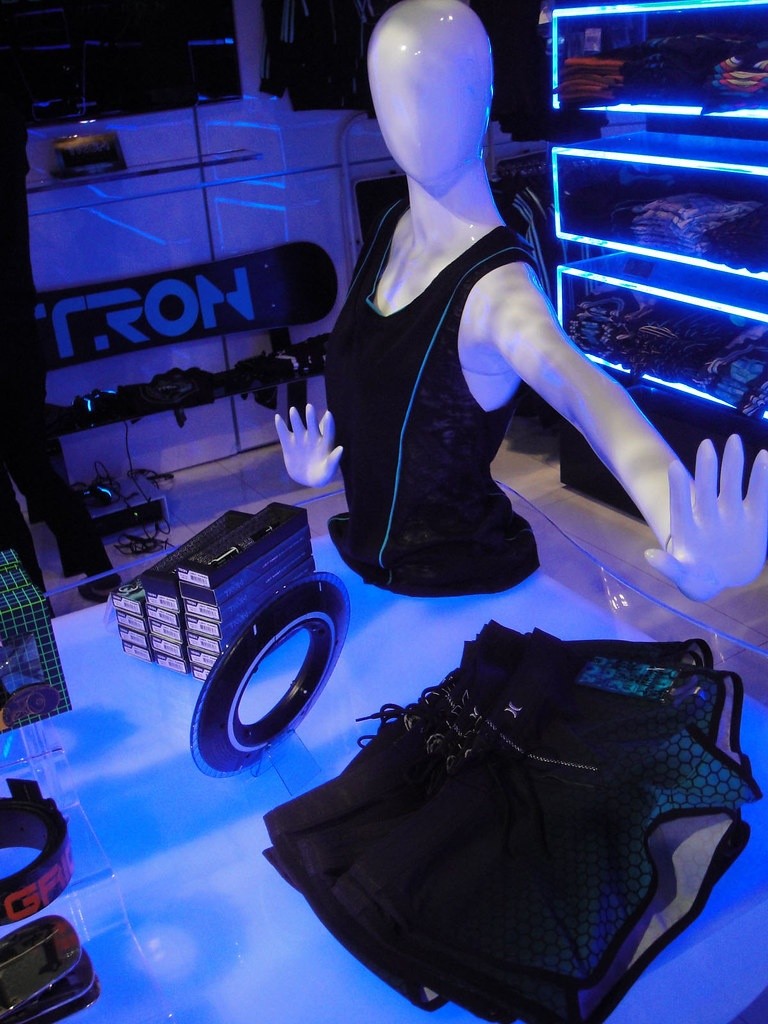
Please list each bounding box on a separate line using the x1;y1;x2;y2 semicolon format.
275;0;767;603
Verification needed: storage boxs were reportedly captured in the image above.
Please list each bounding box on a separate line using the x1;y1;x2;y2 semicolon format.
111;501;316;683
0;548;73;734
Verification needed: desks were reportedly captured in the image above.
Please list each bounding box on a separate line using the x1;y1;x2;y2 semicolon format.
0;535;768;1024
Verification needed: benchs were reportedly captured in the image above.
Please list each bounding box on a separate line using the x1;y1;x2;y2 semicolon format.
32;236;337;476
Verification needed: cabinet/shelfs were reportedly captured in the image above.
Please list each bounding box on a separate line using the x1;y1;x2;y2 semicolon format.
548;0;768;527
